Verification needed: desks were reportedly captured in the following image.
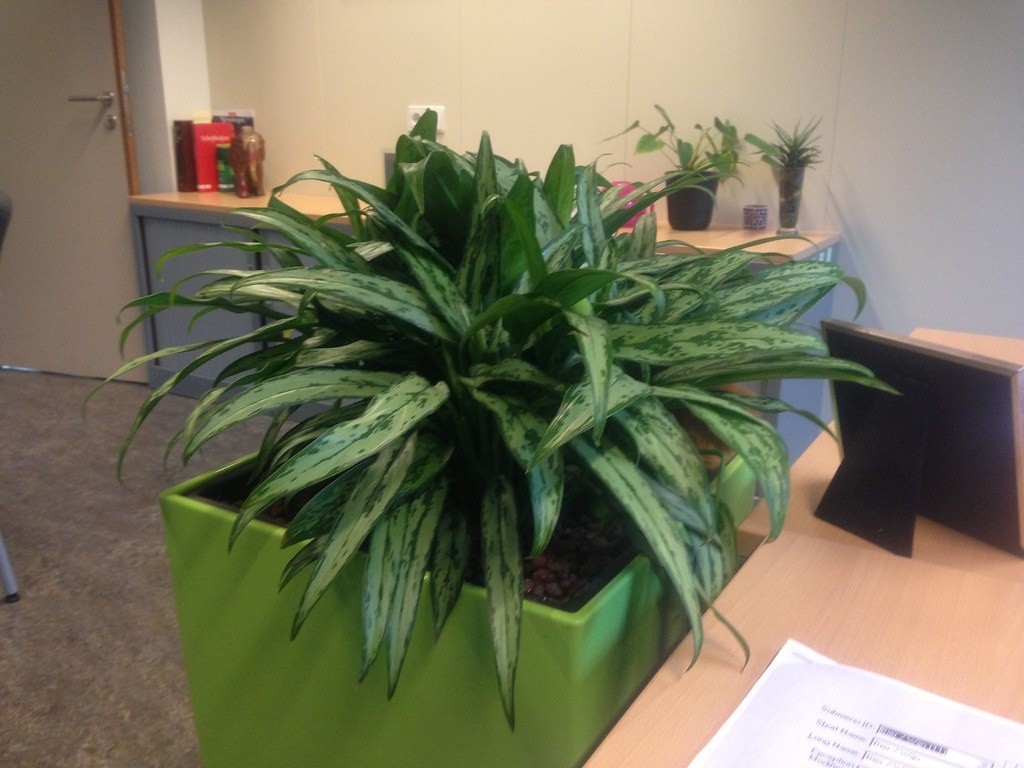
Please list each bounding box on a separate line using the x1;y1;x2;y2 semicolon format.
581;320;1024;768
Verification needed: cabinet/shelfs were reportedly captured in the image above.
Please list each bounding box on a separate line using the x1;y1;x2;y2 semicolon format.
128;193;844;496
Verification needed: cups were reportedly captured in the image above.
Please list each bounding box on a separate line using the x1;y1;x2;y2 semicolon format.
743;204;768;230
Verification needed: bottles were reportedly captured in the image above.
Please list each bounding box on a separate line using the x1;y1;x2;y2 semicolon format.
229;126;266;197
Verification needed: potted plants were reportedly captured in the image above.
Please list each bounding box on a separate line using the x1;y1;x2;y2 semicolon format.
757;119;826;238
83;112;905;768
604;102;747;230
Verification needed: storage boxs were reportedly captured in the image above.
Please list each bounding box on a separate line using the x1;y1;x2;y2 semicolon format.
176;117;237;194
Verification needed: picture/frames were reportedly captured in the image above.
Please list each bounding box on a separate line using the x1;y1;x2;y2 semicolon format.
819;319;1023;557
